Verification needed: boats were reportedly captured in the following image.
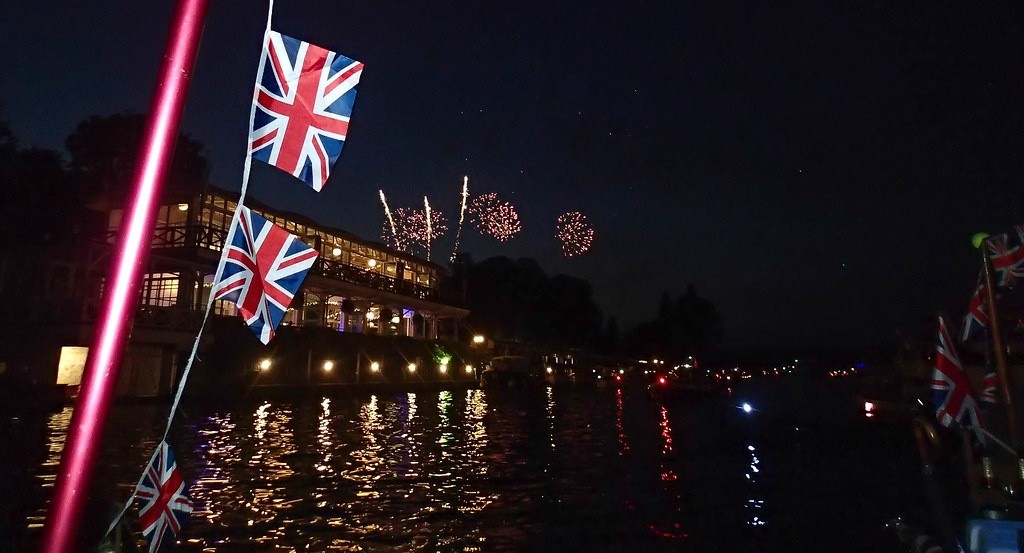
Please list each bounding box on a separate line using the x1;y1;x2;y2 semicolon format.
482;356;526;386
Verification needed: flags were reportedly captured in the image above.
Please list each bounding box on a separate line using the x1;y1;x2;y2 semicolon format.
931;225;1024;448
214;204;319;345
252;30;364;192
137;440;196;553
